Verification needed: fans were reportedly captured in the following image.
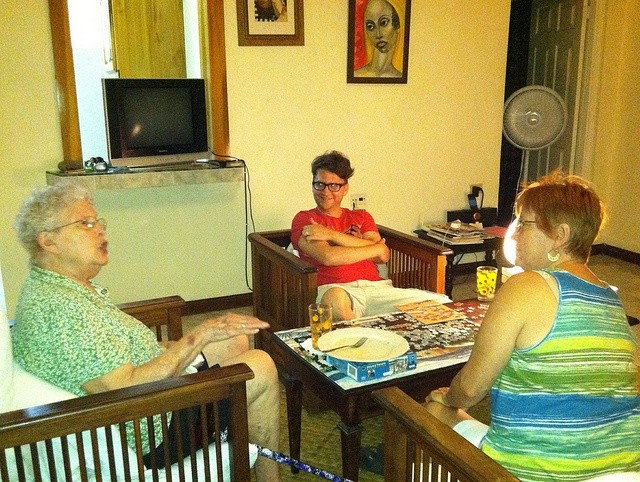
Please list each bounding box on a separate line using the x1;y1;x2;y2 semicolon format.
503;85;568;223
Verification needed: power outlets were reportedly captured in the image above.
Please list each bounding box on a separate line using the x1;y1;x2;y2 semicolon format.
471;182;484;198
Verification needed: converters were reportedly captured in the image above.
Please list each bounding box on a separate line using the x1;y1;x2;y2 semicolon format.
473;185;481;197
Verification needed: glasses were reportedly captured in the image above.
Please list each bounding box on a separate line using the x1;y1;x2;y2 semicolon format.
311;181;347;192
47;216;107;234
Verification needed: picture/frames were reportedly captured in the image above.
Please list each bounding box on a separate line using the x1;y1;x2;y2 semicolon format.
346;1;412;85
236;0;305;47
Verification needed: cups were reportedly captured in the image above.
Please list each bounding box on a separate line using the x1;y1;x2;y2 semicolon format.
476;265;499;302
308;302;333;351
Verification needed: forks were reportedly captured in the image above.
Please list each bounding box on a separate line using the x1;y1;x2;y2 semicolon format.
321;336;368;354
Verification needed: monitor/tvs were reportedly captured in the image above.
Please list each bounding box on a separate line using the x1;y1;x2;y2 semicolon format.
100;77;211;172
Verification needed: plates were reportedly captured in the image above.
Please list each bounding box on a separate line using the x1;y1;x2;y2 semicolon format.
317;326;410;363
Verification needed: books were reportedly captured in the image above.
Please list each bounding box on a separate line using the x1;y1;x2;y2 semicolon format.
427;222;485;248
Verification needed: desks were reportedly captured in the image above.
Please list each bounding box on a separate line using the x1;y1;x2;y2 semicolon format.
414;223;509;300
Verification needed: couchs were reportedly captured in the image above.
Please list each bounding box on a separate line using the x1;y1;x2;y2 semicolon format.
369;385;520;482
0;294;256;482
248;224;455;416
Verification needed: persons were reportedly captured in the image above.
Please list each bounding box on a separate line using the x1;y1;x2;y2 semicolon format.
423;171;640;482
289;150;451;322
8;178;280;481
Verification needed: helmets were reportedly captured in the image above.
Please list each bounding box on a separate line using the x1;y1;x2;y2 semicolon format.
514;219;538;232
